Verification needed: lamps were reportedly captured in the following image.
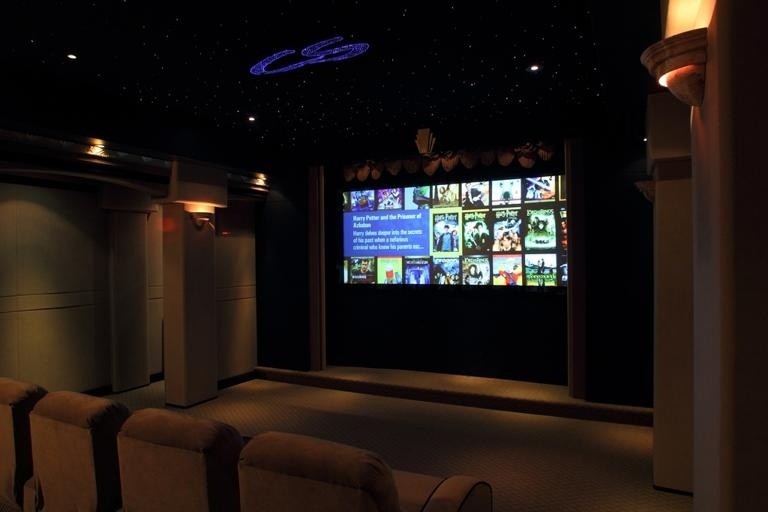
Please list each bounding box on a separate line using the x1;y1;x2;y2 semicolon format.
639;27;708;111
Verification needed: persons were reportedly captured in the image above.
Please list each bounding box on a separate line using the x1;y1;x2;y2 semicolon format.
465;265;482;285
434;225;458;252
542;259;544;273
538;261;541;273
464;182;483;209
351;259;375;284
467;223;489;250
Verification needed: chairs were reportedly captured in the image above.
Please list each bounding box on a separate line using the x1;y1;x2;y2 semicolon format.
0;376;493;512
